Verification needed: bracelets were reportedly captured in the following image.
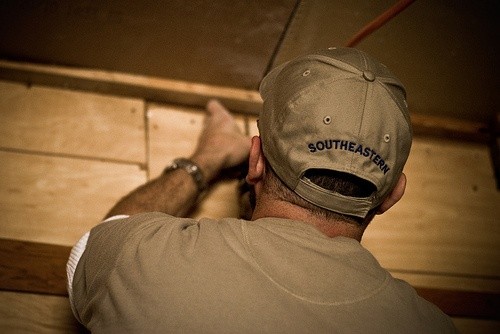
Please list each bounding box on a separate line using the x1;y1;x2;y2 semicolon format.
162;156;207;203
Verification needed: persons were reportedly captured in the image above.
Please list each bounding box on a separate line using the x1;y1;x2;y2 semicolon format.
64;46;467;334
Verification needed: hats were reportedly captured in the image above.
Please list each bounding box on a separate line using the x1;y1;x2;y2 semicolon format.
259;46;414;219
